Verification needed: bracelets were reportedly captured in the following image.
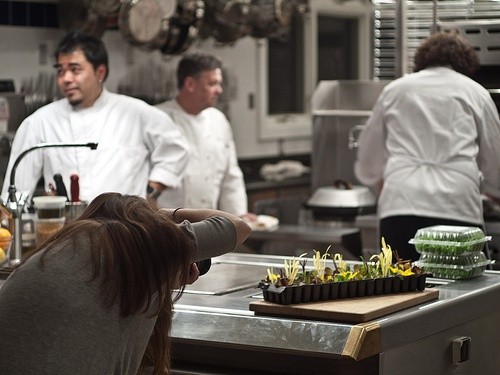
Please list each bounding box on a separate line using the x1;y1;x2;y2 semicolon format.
172;207;183;219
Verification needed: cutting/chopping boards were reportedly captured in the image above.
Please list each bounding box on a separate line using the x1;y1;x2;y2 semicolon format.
249;289;440;322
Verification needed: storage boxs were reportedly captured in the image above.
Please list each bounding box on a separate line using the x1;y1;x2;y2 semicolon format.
409;224;496;280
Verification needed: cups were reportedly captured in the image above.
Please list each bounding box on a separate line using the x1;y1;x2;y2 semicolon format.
21;196;88;248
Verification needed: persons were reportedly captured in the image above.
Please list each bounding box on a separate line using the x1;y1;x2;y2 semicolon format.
152;52;257;222
353;31;500;264
0;192;252;375
0;32;191;205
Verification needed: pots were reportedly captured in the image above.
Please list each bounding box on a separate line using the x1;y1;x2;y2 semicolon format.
64;0;311;61
303;179;377;223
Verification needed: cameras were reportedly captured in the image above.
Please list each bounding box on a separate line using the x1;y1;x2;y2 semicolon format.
196;258;211;275
